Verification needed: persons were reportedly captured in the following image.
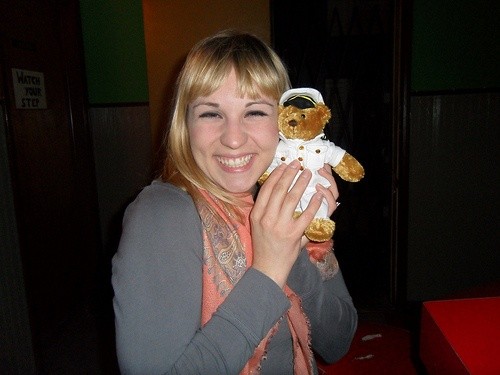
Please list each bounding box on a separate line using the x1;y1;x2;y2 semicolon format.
111;28;359;375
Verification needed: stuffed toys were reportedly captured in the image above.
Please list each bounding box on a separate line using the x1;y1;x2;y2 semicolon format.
257;87;364;244
